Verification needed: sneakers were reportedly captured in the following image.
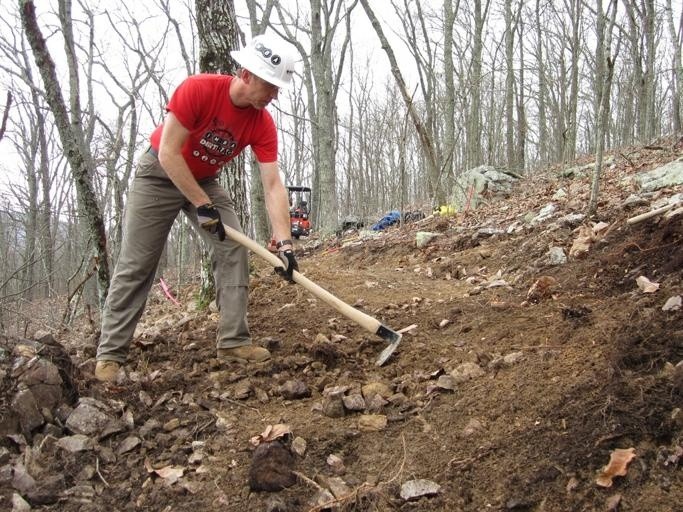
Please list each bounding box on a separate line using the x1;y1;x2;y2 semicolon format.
95;360;121;381
217;345;271;363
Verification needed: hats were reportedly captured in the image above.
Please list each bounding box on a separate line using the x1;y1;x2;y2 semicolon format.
230;35;295;89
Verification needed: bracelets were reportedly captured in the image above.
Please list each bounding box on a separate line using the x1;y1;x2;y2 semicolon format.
276;239;293;249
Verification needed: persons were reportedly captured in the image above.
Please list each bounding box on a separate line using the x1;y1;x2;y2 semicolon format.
95;34;299;384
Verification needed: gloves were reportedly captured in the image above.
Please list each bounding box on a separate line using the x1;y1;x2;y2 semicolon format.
197;203;225;241
274;249;300;284
